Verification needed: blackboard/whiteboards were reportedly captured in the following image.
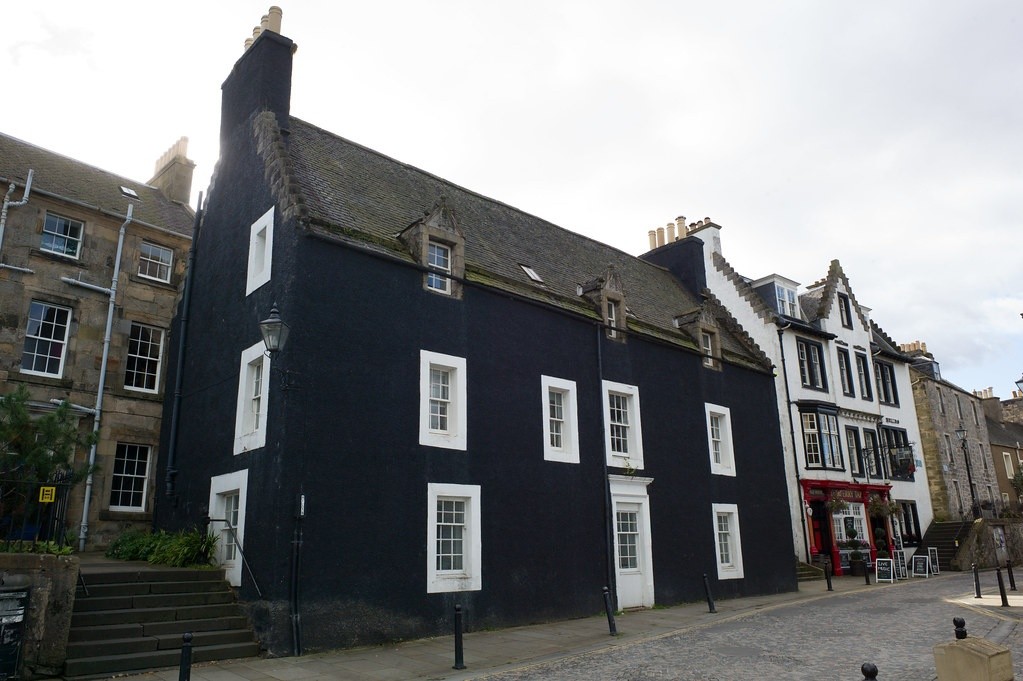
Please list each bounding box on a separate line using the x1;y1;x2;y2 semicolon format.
928;547;940;575
911;555;929;576
875;558;893;581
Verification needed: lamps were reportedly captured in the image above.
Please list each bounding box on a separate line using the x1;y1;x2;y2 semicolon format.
890;447;898;456
257;300;292;360
